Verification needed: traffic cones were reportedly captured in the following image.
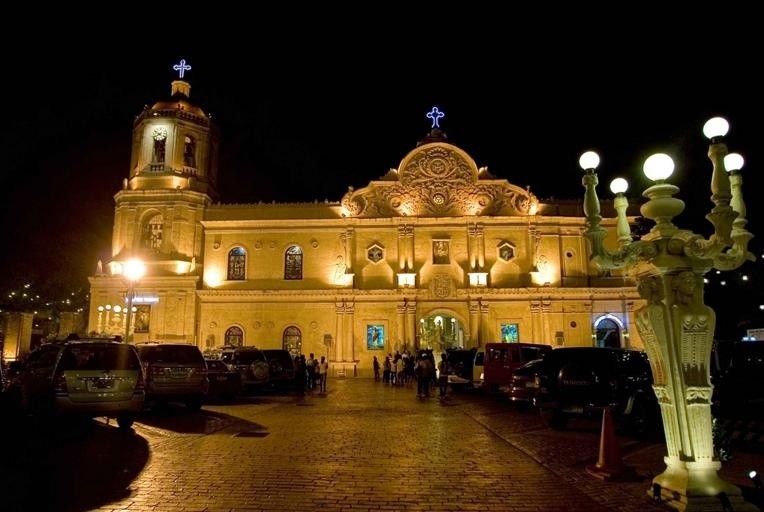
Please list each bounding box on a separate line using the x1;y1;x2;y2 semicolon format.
586;407;630;481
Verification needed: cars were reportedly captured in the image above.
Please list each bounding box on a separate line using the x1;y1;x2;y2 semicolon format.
445;344;663;432
5;334;295;427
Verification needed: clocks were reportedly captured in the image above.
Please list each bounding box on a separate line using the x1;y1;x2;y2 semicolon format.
152;126;168;142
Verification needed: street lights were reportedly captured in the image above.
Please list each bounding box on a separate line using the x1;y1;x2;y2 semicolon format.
579;117;756;512
123;258;145;344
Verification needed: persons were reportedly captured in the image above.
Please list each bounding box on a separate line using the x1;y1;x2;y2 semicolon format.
373;349;412;388
413;352;433;398
437;352;450;396
318;356;328;393
306;352;315;389
